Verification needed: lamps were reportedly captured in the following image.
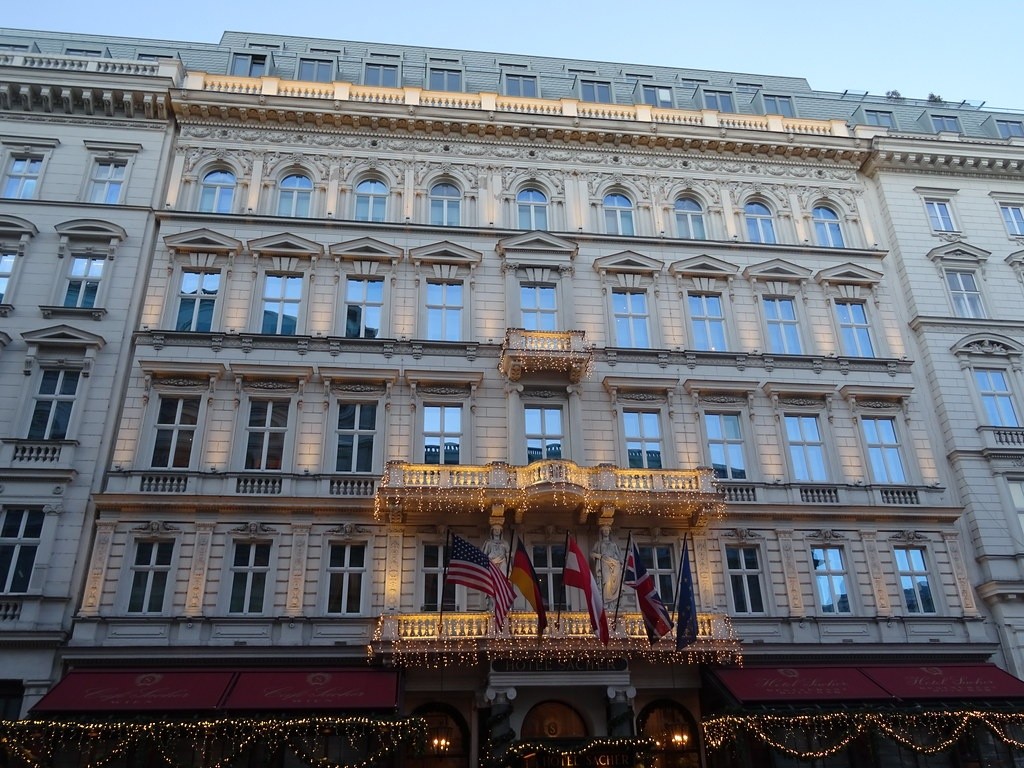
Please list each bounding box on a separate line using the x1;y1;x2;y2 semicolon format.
608;680;638;706
664;717;691;748
484;686;517;704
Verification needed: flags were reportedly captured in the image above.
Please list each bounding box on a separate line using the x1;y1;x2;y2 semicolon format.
509;536;548;647
562;536;609;648
623;539;674;647
446;534;517;632
676;542;698;654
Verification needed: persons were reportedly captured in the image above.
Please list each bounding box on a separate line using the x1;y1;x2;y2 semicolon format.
481;524;513;578
590;525;624;611
